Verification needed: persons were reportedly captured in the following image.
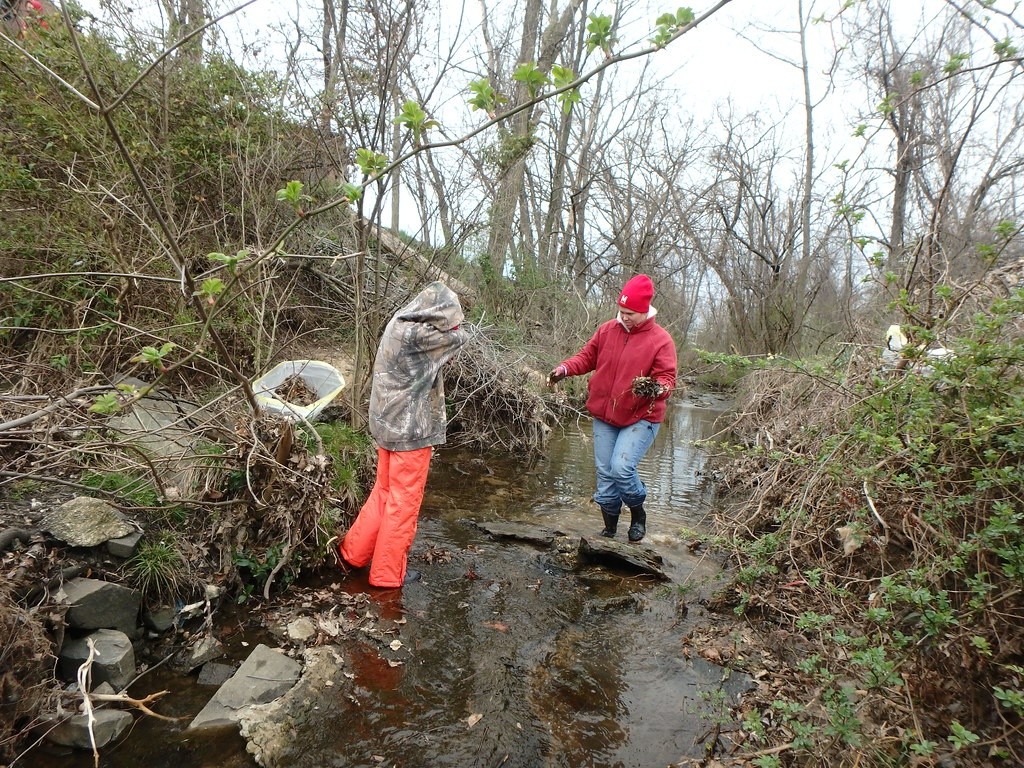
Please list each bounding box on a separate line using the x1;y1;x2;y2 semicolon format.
547;273;679;544
339;279;468;588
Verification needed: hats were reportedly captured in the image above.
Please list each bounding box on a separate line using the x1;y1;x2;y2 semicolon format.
617;274;654;313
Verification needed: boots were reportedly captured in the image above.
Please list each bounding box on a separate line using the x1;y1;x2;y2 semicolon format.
628;504;646;542
600;509;619;537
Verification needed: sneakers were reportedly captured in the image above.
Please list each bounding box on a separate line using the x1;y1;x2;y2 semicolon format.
326;547;370;575
402;569;422;585
